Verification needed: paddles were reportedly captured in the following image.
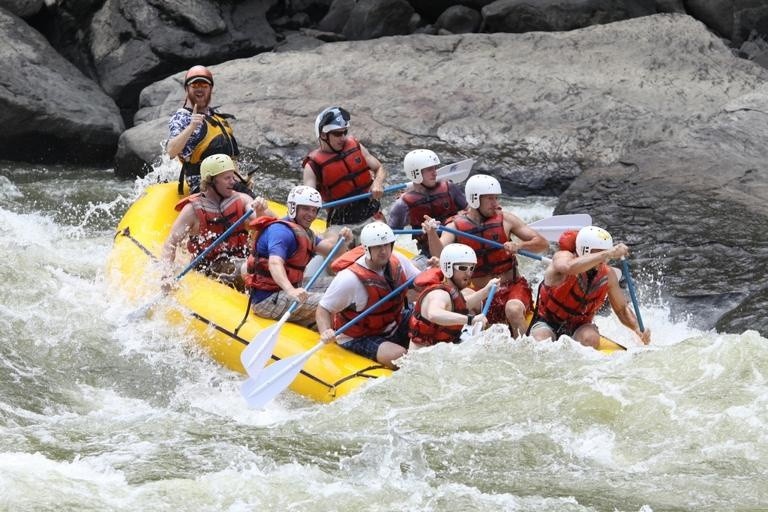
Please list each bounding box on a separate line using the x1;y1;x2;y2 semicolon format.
390;213;592;251
279;157;479;222
433;222;623;285
239;233;348;380
235;271;421;412
121;205;256;325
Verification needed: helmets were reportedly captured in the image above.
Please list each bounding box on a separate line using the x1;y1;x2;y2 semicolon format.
576;227;612;268
314;107;351;139
360;221;395;260
439;244;479;277
200;153;235;181
288;185;324;216
185;65;213;86
404;150;441;185
465;174;503;209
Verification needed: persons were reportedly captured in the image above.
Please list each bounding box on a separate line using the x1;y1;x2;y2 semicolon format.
387;149;466;259
422;173;550;340
408;243;501;353
243;185;354;330
166;65;256;198
315;221;421;369
158;153;274;296
302;106;386;248
529;227;652;351
